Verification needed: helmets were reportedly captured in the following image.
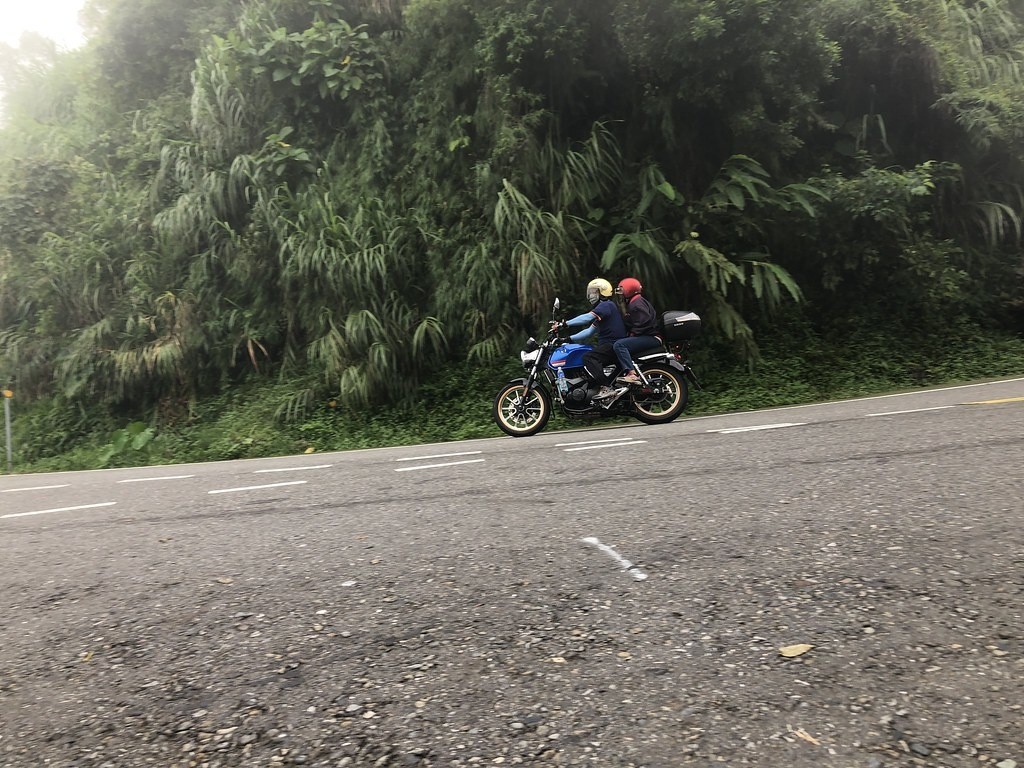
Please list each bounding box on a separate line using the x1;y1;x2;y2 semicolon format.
618;278;644;298
588;277;613;297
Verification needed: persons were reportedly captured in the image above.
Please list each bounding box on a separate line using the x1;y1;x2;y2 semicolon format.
614;278;663;385
552;279;628;399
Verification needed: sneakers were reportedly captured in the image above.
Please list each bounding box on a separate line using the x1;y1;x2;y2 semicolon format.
616;373;643;386
591;385;618;400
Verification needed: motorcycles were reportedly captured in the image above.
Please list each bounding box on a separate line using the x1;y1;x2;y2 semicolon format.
492;297;703;438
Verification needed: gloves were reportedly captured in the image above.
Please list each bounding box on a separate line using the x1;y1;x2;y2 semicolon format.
559;337;567;343
551;322;564;333
